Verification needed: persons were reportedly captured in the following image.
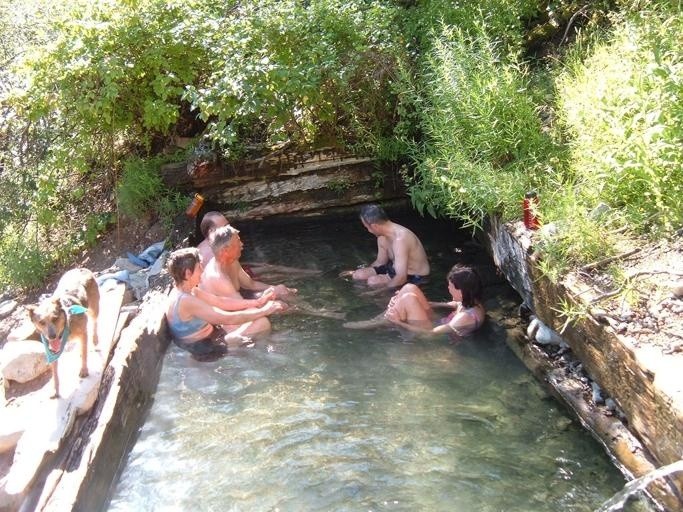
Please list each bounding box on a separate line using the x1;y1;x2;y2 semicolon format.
195;224;298;311
195;211;322;282
341;262;486;343
338;203;431;298
165;247;284;362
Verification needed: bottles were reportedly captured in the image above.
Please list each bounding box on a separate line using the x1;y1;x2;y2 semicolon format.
524;191;541;229
185;192;204;219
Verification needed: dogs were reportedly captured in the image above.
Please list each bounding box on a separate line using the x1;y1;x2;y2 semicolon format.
22;268;102;399
